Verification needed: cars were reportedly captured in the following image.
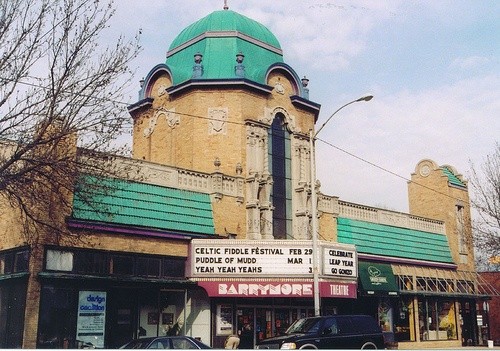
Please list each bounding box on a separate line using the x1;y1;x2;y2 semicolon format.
113;335;213;349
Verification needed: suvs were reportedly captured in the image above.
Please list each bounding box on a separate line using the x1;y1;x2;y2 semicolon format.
257;313;386;350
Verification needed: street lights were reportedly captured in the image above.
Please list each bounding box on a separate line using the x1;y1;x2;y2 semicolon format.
309;94;375;317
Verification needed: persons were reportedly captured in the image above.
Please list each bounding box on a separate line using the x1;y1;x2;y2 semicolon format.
237;327;253;349
224;335;240;350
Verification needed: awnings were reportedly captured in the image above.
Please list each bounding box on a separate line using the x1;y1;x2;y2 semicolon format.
358;261;399;298
